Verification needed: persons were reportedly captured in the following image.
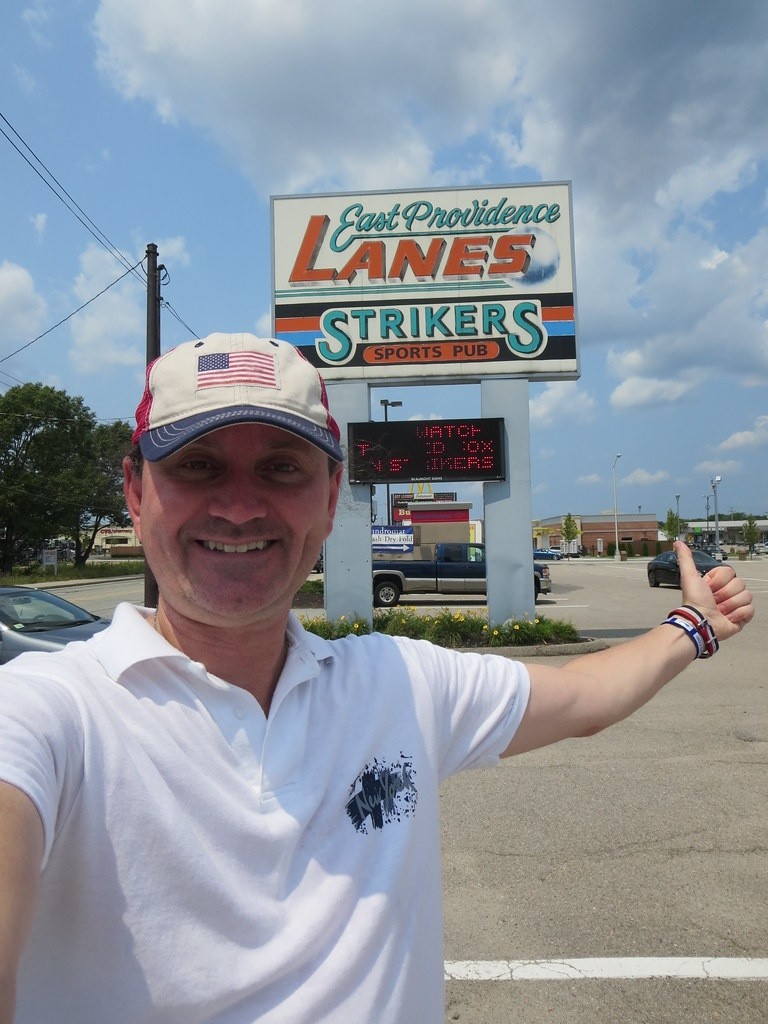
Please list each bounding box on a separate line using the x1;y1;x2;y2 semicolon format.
2;334;754;1023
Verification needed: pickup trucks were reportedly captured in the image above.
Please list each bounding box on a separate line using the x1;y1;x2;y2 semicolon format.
372;542;552;608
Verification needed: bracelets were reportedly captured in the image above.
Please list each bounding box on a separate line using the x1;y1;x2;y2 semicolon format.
662;605;720;661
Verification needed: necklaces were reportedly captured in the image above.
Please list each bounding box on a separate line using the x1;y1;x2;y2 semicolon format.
151;608;162;635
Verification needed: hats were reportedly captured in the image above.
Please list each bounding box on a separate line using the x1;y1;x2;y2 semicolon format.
131;331;346;469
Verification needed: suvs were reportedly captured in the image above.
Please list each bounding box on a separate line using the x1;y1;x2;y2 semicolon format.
543;546;569;558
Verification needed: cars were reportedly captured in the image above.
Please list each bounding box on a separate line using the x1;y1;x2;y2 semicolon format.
0;584;112;667
14;536;88;562
647;550;726;587
533;549;563;561
686;539;768;560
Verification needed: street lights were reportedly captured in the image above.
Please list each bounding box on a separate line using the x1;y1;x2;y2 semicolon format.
380;399;402;526
676;494;681;540
612;454;621;562
638;505;641;513
702;494;714;539
